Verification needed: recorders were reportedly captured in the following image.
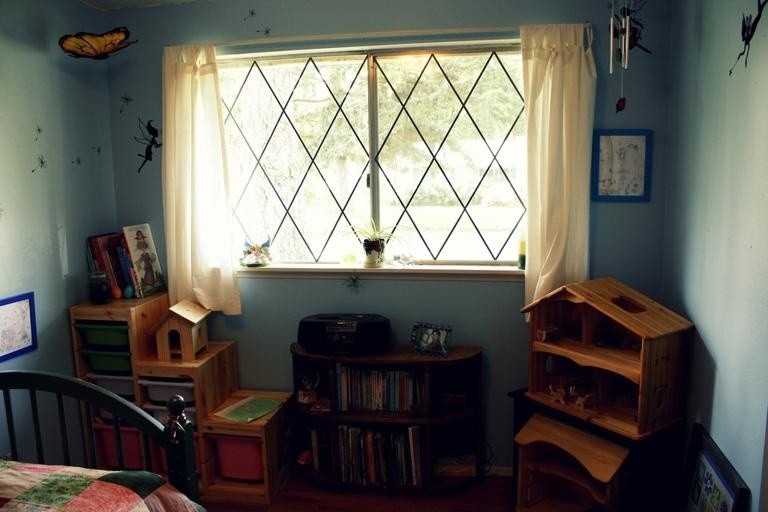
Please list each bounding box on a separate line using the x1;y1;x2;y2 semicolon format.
297;313;392;358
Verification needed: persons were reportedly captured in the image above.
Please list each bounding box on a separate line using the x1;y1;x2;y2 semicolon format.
416;332;430;348
427;329;443;349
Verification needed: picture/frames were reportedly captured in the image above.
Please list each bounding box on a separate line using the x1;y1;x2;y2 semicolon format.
591;127;654;203
1;291;38;363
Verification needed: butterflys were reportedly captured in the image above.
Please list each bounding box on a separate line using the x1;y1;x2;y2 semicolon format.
58;26;139;60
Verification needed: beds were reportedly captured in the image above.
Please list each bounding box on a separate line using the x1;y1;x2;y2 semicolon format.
0;369;201;512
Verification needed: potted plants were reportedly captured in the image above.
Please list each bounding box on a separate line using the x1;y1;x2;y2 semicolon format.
344;215;412;267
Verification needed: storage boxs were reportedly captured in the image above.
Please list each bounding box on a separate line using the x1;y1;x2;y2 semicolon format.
75;323;198;474
206;432;264;481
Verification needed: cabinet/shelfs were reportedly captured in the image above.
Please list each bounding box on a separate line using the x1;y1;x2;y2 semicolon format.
287;341;483;494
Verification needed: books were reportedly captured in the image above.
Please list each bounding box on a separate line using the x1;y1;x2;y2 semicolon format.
310;397;331;412
332;360;478;490
85;223;168;299
